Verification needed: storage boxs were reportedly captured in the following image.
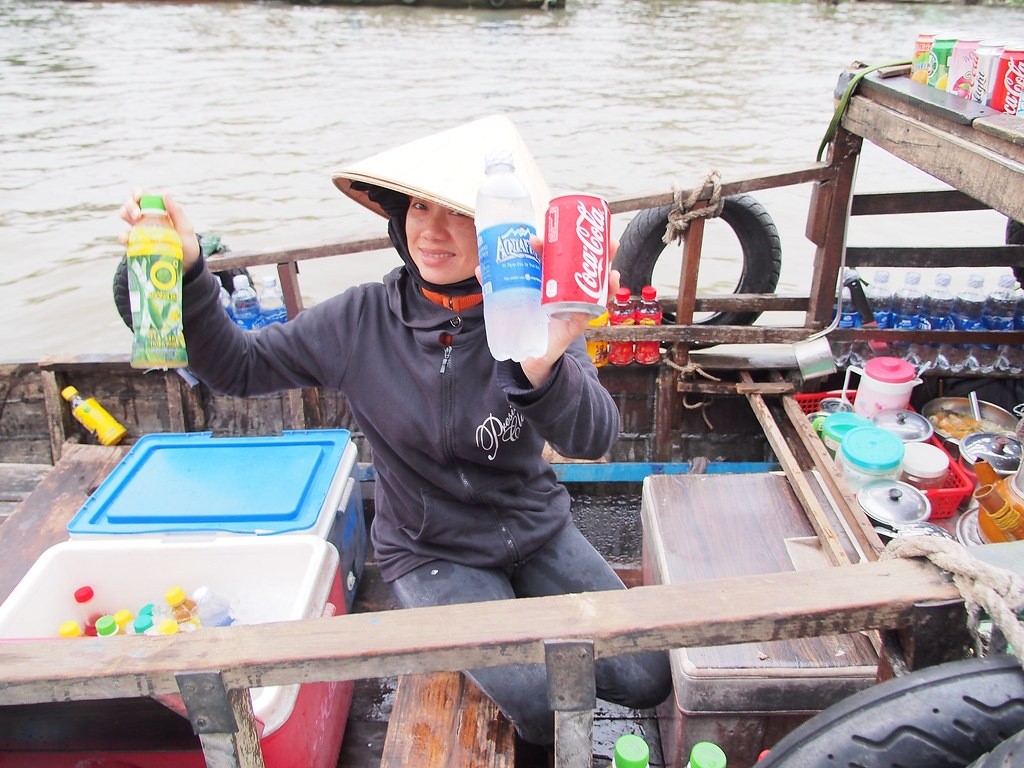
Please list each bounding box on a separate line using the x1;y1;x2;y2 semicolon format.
638;466;878;768
0;429;368;768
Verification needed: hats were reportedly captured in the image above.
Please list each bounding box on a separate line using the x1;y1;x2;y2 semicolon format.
331;114;549;243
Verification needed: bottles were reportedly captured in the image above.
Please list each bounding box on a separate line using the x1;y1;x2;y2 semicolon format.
474;151;548;361
58;581;237;639
125;194;188;369
587;286;662;368
213;274;287;332
684;741;727;768
606;734;650;768
830;265;1024;377
61;386;127;447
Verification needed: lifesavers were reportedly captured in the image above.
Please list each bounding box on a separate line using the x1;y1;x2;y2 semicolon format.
606;192;784;348
112;235;255;334
751;654;1023;768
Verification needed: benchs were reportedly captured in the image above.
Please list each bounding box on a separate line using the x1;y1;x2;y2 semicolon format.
0;445;133;610
380;617;517;768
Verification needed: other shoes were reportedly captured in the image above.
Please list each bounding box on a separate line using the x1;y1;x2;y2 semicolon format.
518;734;553;768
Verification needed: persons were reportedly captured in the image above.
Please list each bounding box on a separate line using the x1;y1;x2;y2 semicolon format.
119;116;671;768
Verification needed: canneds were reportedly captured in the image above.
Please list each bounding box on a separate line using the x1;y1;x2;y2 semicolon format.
542;194;611;319
910;29;1024;118
896;442;948;491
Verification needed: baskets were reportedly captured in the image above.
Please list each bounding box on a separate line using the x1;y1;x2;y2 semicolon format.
795;390;974;517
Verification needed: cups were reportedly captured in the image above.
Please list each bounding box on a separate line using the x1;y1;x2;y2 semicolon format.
942;431;1024;513
807;397;933;442
857;478;931;543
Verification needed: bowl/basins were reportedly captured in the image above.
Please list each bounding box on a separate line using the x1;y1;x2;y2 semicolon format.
923;397;1020;458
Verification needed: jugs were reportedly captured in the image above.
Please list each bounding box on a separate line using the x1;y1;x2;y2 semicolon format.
844;355;923;418
